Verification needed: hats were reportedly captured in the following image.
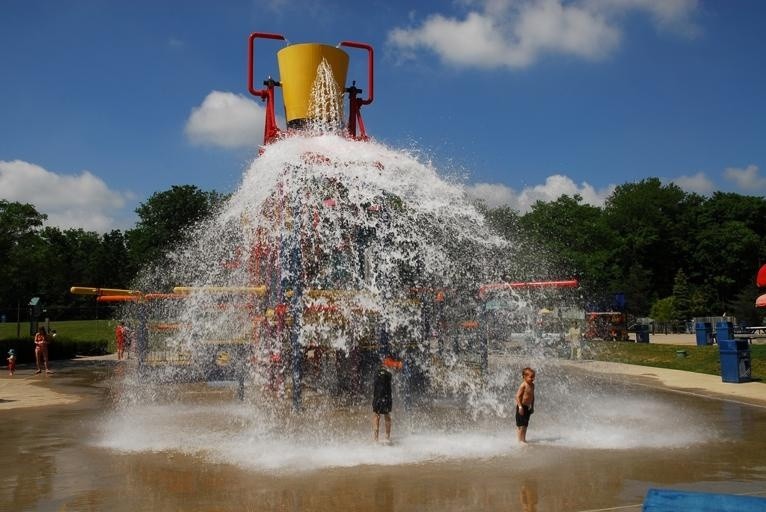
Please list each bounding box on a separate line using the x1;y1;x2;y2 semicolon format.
8;349;15;355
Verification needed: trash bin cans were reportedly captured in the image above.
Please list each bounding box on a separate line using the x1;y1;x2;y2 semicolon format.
719;340;751;383
716;322;734;345
635;324;649;343
695;322;713;345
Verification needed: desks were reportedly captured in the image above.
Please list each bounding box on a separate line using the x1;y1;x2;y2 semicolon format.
745;327;765;344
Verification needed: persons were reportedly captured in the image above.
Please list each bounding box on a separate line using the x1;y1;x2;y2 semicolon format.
518;469;541;511
515;367;534;443
567;320;583;363
371;361;392;445
33;326;54;374
6;349;16;376
115;317;148;360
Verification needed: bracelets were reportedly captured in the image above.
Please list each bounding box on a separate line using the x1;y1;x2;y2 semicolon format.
518;406;522;409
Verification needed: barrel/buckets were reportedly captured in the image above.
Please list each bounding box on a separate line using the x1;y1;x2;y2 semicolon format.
276;42;349;125
